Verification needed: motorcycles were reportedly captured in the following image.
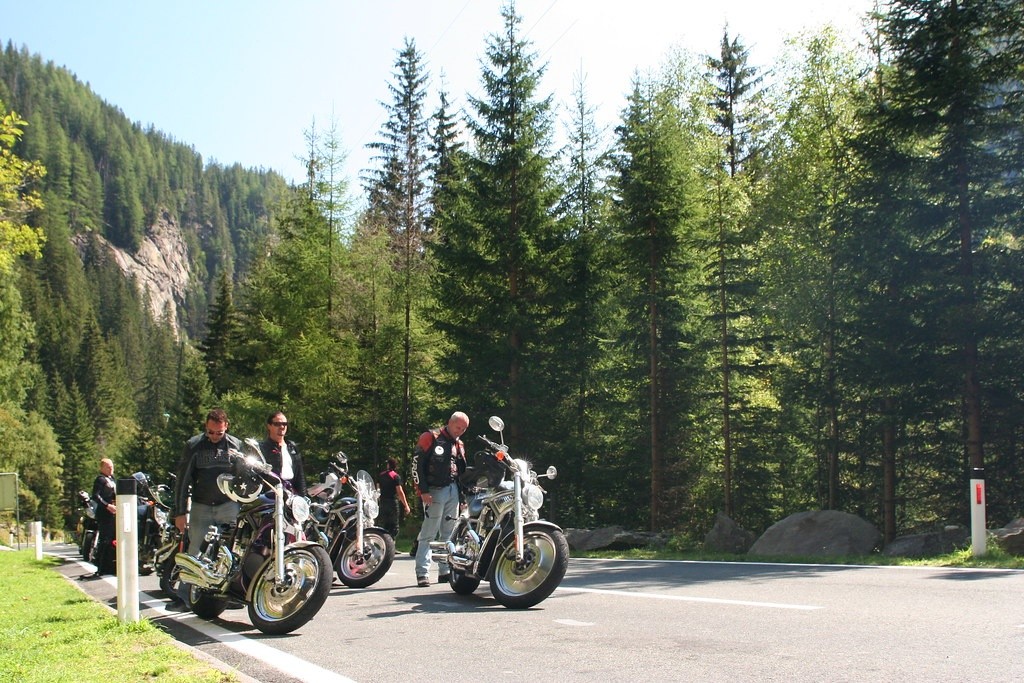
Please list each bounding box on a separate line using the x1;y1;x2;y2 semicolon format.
308;449;397;590
77;492;103;561
172;437;335;637
128;471;175;575
428;415;571;610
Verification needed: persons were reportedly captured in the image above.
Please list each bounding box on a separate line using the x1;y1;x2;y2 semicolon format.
407;410;473;587
251;409;309;523
92;457;122;579
370;453;411;555
164;407;247;613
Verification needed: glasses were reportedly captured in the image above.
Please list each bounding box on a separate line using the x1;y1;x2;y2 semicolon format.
207;426;225;436
270;422;288;427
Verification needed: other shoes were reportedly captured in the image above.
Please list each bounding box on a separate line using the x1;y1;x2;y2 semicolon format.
417;575;450;586
